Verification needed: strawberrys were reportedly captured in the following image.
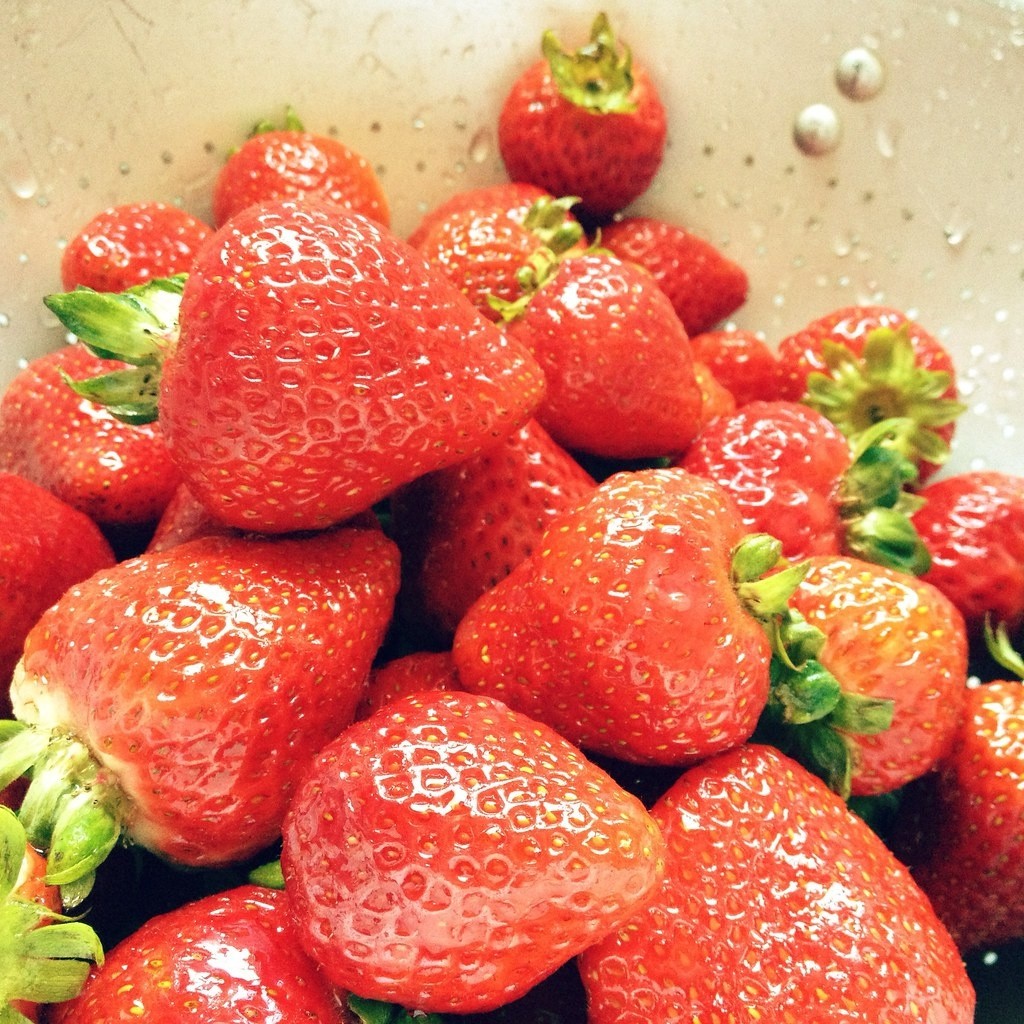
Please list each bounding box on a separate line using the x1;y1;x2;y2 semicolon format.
0;10;1024;1024
